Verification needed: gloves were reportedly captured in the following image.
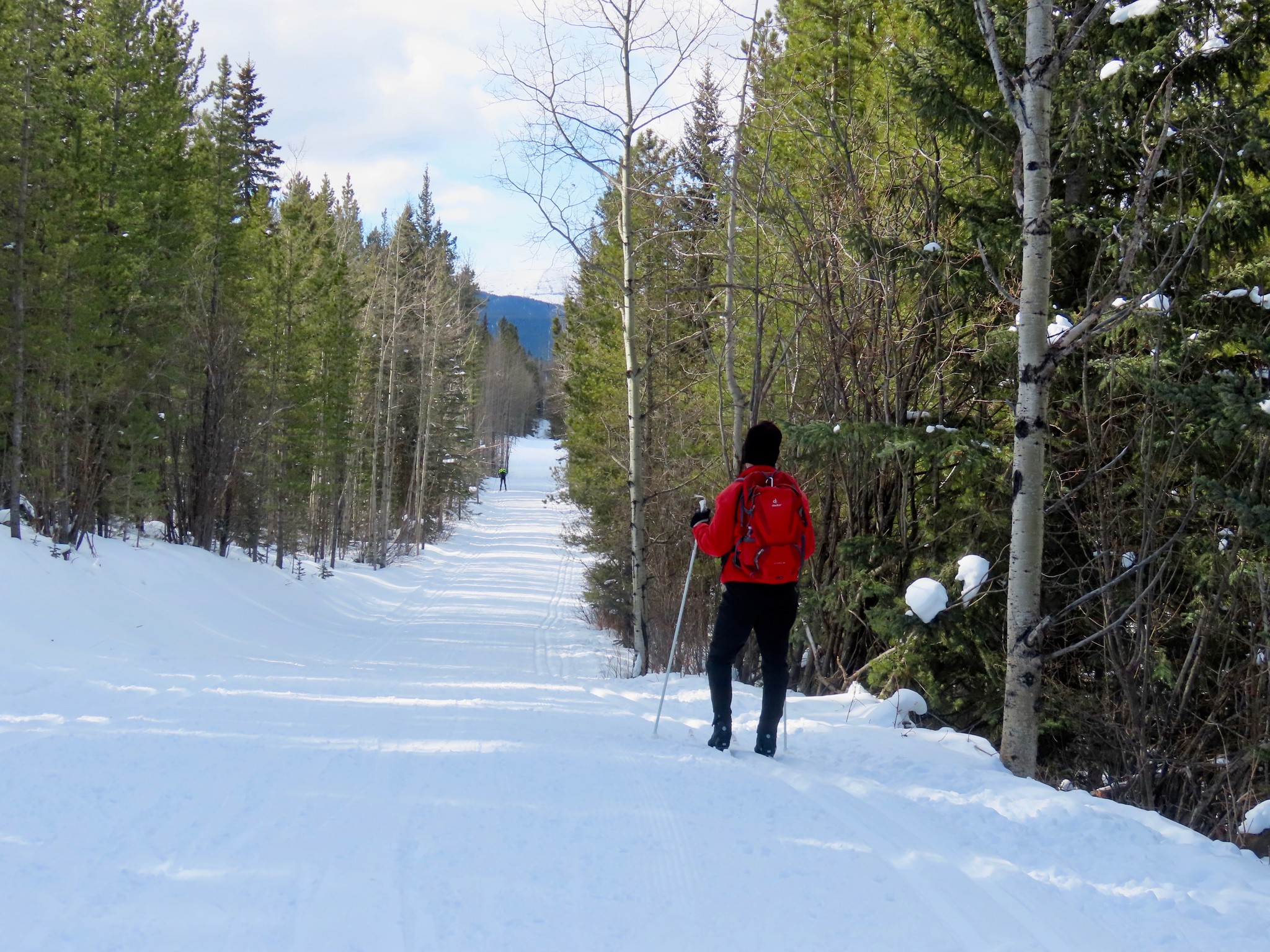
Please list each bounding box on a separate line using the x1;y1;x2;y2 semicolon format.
691;508;711;528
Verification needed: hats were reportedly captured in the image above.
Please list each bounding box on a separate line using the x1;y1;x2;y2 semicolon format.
740;420;783;474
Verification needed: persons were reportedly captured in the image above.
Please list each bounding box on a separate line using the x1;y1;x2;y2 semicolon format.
498;467;507;491
689;421;816;758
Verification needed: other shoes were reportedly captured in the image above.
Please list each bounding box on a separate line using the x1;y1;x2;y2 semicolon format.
708;732;730;750
754;736;775;756
